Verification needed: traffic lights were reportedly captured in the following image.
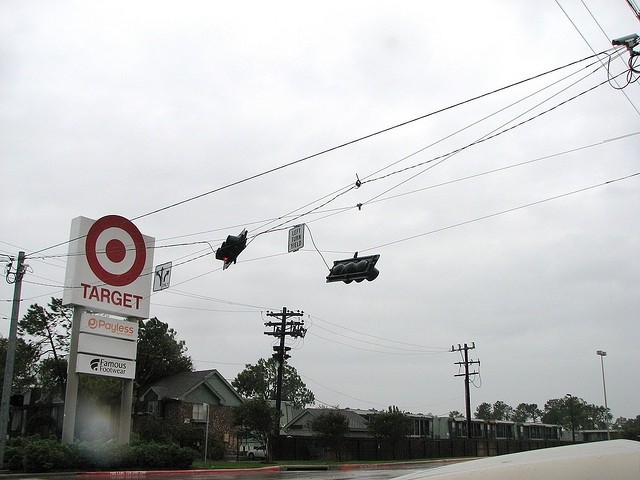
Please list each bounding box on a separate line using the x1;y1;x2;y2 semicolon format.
326;254;380;284
216;234;243;264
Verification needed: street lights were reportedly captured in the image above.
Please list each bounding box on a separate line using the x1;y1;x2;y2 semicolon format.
566;394;574;441
597;351;610;440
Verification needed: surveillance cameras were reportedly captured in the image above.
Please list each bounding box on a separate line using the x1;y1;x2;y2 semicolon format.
610;33;639;58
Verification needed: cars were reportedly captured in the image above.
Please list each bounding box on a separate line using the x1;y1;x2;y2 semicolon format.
247;444;266;460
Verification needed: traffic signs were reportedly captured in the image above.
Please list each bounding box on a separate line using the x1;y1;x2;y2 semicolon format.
288;223;304;252
